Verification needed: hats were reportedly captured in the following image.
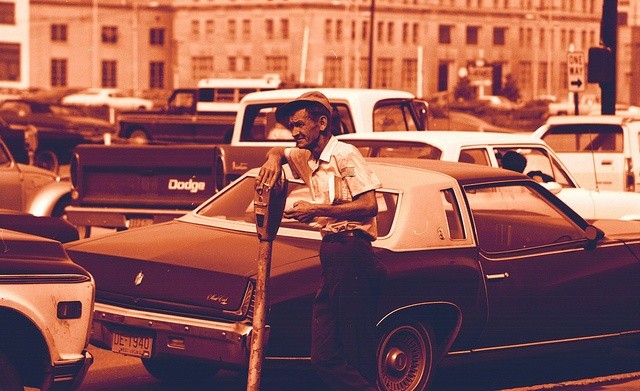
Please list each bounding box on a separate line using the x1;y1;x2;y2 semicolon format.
275;91;333;129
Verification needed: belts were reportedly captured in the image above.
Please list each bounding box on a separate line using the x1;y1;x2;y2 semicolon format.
324;232;365;241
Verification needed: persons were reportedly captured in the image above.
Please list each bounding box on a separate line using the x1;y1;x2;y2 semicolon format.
255;91;380;391
500;151;544;185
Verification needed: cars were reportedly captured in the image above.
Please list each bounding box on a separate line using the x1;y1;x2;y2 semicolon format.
0;139;90;219
0;100;118;134
63;155;640;391
335;131;639;222
0;228;96;391
60;88;153;113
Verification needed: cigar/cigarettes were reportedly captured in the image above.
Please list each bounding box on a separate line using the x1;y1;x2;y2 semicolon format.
263;181;270;187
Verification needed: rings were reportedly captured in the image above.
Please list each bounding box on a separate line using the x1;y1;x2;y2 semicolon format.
265;178;271;184
256;175;262;181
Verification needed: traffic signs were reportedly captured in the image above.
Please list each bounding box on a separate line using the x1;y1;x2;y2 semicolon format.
567;53;585;93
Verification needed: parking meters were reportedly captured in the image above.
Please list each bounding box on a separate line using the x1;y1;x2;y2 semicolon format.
247;167;288;391
24;125;39;166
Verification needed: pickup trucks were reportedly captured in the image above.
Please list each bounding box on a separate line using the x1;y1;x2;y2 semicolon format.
118;89;277;144
64;88;429;226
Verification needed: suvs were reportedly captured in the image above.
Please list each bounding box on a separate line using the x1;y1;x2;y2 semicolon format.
516;115;640;191
